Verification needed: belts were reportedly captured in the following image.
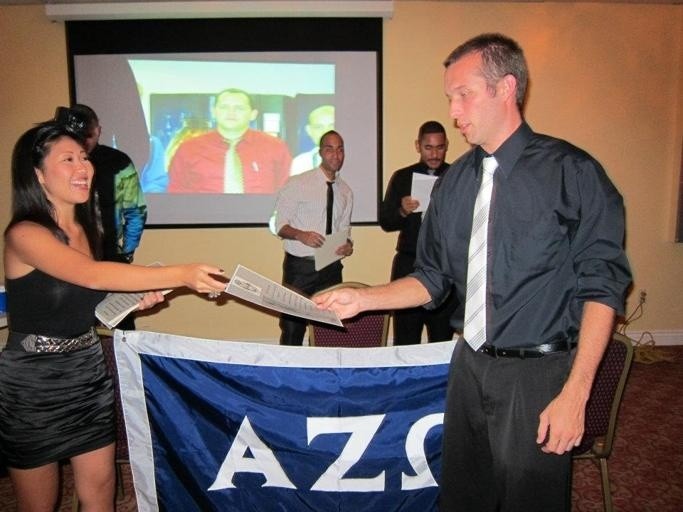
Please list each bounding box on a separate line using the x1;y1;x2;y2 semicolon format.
480;341;580;358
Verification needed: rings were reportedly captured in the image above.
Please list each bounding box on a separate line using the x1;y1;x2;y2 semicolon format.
208;291;221;299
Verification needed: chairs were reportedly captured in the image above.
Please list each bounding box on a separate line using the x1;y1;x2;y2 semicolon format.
573;331;634;512
308;282;390;346
72;326;130;512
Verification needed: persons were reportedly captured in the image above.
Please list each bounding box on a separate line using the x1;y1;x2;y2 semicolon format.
379;120;461;346
70;102;149;330
0;107;231;512
313;31;634;511
165;88;292;192
273;129;354;345
292;104;334;175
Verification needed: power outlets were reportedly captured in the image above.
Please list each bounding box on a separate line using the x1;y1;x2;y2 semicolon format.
639;289;647;303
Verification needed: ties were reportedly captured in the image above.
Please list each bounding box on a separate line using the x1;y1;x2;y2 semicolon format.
463;157;499;354
327;182;334;234
427;170;436;175
223;139;246;195
